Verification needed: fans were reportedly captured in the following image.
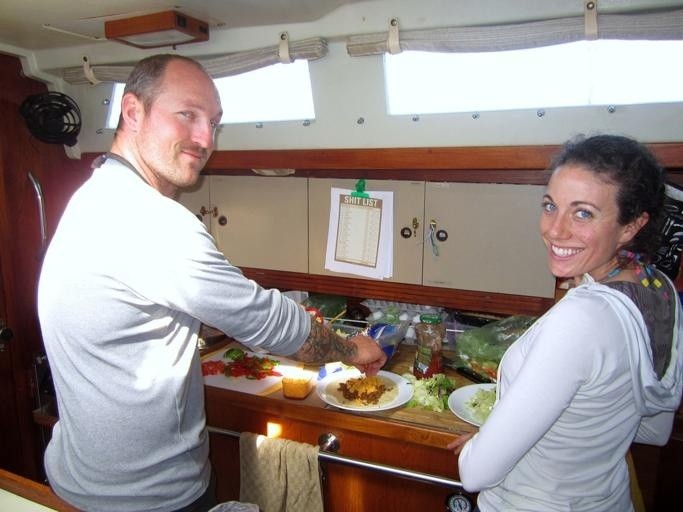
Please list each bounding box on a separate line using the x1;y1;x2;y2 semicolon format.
21;90;84;147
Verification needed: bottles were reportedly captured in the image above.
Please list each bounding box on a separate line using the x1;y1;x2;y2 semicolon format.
413;315;444;380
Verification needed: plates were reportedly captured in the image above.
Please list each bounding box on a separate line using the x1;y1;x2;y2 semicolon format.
448;380;559;427
316;365;414;413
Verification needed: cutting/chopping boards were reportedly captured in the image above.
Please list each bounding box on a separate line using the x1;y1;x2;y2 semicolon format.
190;347;307;396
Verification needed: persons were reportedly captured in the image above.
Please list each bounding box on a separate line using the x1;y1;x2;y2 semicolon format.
448;136;682;508
37;54;387;508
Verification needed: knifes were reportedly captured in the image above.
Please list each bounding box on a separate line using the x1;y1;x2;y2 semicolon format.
441;359;495;384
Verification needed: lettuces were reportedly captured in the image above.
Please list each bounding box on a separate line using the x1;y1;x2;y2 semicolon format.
414;374;447;413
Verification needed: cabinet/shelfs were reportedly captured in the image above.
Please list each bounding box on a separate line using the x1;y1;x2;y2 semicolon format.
171;174;308;276
205;404;539;512
308;177;555;299
6;67;108;474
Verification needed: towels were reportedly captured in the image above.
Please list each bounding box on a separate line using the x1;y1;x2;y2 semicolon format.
240;431;324;512
